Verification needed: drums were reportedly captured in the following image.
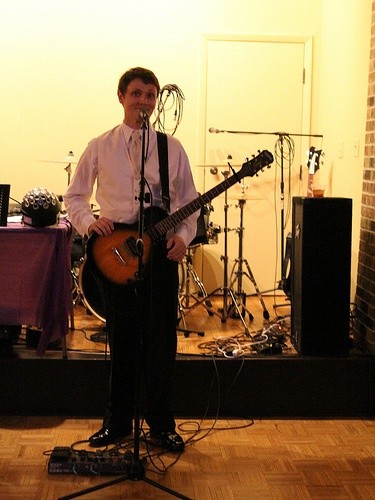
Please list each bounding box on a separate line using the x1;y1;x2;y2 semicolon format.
187;191;209;249
78;248;188;325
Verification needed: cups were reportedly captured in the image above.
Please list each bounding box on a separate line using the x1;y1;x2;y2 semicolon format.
312;185;325;197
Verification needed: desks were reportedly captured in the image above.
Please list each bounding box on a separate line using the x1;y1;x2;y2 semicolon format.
0;219;74;359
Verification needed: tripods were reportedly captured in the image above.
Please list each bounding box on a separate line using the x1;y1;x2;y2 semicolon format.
58;119;195;500
177;172;270;338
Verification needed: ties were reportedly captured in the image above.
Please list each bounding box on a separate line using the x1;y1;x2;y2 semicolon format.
130;131;142;177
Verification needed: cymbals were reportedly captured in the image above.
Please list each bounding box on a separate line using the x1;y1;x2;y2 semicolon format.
34;154;82;166
194;162;243;169
228;195;267;202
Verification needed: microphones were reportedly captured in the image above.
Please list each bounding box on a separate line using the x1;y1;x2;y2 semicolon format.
140;109;149;119
209;128;219;133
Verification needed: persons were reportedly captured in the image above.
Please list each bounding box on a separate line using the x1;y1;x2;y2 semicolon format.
63;67;201;452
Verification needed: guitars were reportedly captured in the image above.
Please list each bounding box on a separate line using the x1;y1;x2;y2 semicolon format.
84;146;275;286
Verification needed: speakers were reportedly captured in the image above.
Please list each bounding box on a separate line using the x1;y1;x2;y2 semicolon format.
290;196;352;356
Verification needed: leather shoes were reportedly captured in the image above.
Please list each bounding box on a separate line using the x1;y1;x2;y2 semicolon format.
150;425;185;452
89;424;133;447
